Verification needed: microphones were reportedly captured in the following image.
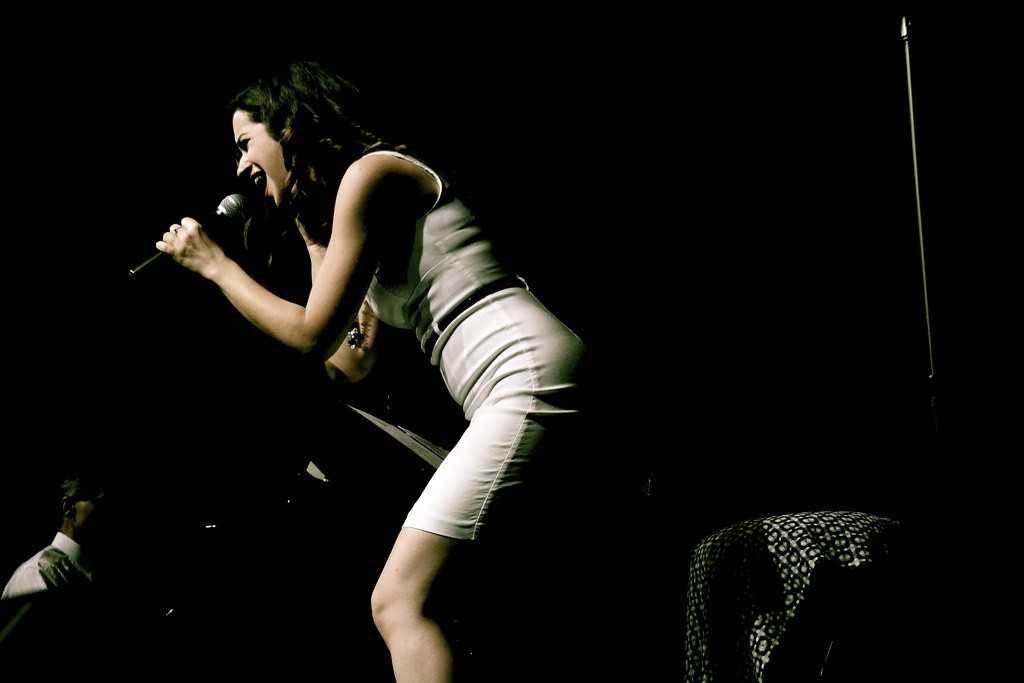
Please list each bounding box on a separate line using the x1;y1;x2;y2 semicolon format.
129;194;249;281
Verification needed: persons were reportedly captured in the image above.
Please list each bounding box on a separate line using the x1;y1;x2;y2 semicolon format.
0;471;128;600
156;62;589;683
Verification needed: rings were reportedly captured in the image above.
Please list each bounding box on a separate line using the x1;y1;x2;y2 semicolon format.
173;226;181;233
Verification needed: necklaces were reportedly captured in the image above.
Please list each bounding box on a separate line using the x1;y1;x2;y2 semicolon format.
347;300;373;354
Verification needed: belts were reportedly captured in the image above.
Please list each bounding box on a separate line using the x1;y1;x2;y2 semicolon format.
421;274;526;360
